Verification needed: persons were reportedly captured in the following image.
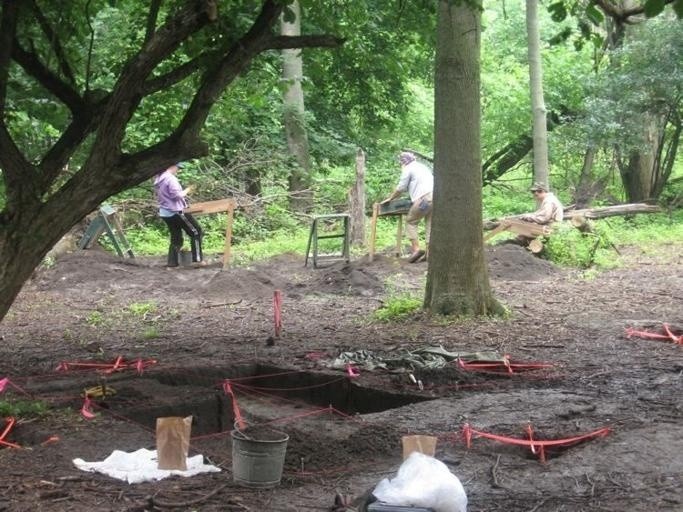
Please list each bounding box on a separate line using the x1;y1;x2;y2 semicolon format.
514;181;563;247
153;160;211;272
380;152;434;263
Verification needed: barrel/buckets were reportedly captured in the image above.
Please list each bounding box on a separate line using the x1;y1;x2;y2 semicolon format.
230;420;289;489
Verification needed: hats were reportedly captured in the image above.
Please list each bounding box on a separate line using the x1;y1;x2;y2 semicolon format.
176;163;185;169
531;182;546;192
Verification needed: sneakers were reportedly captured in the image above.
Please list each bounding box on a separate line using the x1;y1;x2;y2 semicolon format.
410;250;427;263
